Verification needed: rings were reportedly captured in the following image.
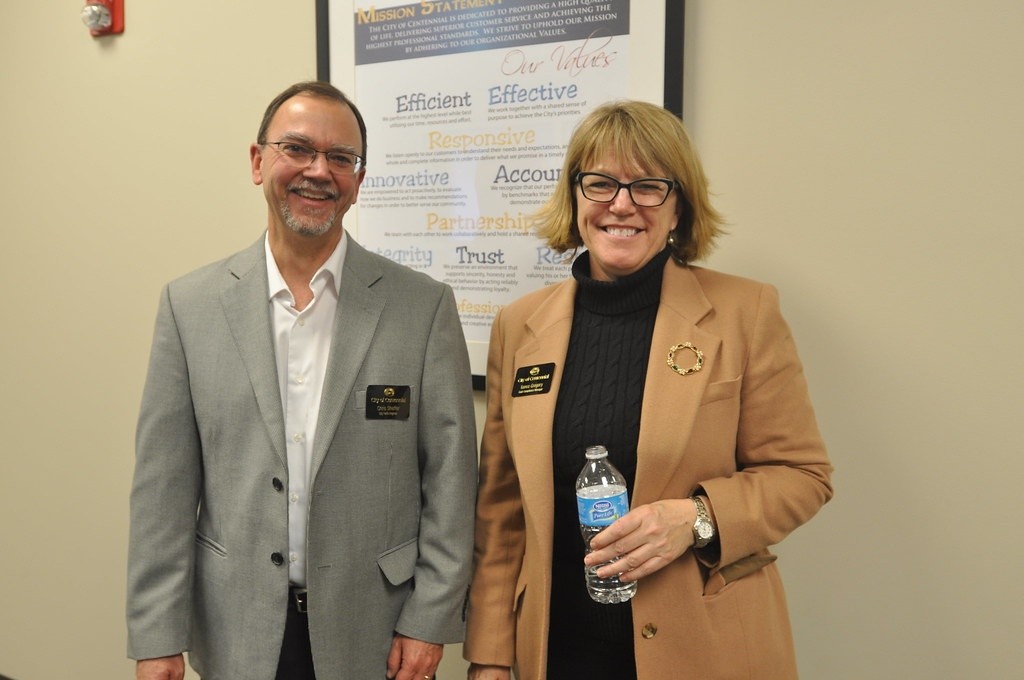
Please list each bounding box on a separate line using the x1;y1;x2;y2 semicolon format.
424;675;430;680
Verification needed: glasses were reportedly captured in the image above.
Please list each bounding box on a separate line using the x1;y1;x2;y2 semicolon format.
571;170;681;207
262;141;367;176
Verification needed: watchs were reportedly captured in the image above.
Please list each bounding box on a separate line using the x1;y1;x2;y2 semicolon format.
689;496;714;548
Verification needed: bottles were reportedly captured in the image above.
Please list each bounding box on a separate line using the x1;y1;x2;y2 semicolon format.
574;446;639;604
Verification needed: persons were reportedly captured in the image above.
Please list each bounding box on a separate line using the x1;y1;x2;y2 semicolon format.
124;81;480;680
462;102;834;680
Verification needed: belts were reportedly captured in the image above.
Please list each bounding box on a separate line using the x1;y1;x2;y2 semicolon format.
287;587;308;613
